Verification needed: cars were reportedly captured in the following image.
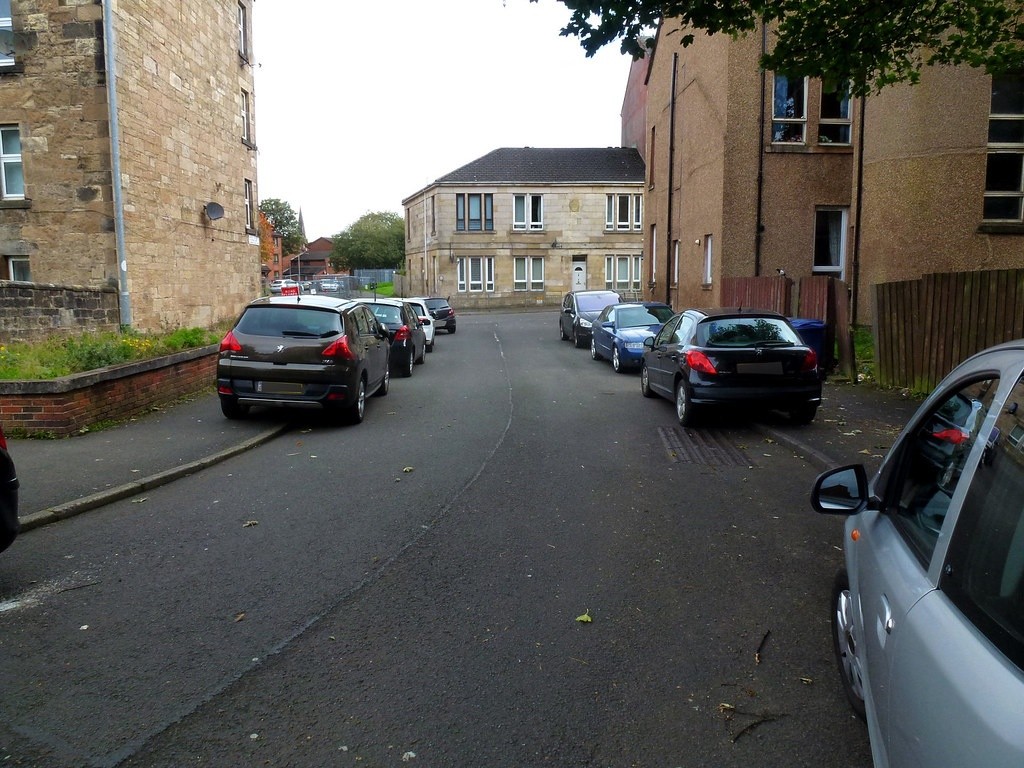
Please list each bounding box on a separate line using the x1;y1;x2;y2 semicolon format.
383;296;435;354
215;294;391;424
558;287;627;347
809;334;1024;768
640;296;823;429
271;276;344;294
589;300;676;374
350;297;426;377
422;297;457;335
